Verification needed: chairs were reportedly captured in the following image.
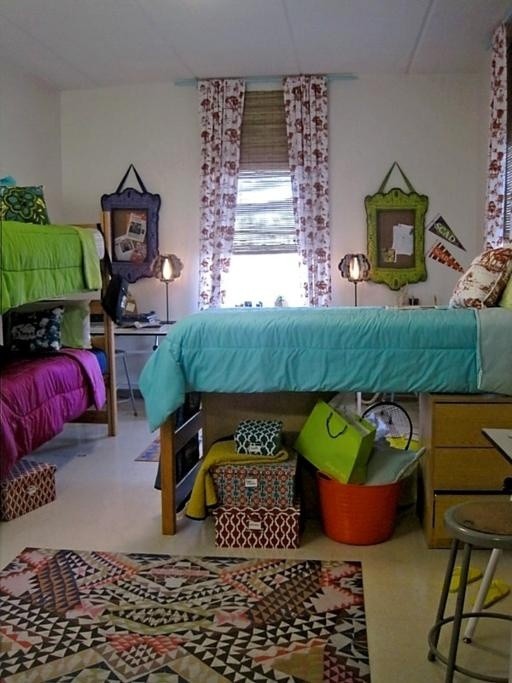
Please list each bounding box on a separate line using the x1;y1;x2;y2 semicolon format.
90;331;138;418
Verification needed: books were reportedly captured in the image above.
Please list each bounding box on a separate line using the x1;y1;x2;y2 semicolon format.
116;324;165;329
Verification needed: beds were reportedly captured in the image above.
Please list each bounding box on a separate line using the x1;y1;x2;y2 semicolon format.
1;209;115;458
158;307;511;534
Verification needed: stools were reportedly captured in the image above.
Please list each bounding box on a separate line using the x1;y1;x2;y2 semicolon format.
425;500;511;681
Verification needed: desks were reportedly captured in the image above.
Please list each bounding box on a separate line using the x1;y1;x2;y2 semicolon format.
462;427;512;642
89;320;173;354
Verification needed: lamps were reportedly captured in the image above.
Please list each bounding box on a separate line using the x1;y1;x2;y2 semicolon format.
338;252;371;306
152;253;184;323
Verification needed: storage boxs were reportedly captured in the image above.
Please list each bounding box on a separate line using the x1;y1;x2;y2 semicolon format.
207;418;302;549
2;459;59;522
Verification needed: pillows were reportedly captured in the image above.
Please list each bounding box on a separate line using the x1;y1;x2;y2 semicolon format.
1;181;51;225
447;242;511;308
3;298;93;353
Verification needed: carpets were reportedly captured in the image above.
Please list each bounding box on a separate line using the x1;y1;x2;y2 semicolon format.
0;545;371;682
133;433;203;462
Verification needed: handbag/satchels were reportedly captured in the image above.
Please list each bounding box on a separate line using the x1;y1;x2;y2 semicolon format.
293;396;376;484
100;273;129;326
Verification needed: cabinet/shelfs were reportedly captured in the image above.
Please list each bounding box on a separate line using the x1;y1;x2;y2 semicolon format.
418;390;511;548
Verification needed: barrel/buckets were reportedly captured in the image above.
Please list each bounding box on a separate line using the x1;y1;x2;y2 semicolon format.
317;469;403;546
